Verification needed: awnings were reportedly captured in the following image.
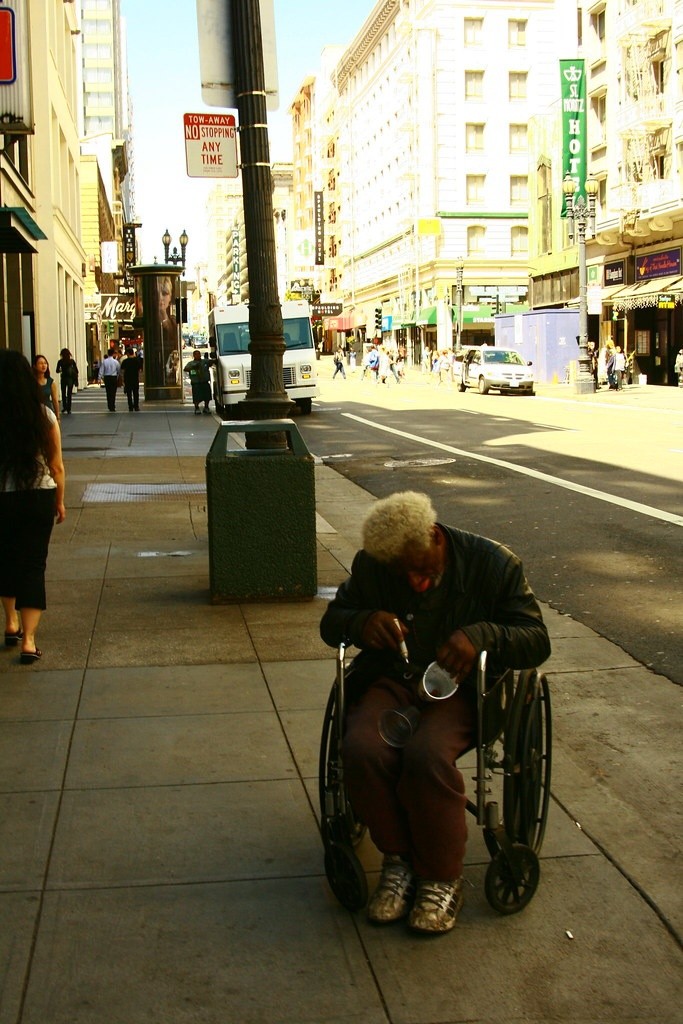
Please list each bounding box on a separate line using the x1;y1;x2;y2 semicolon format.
415;306;436;326
575;275;683;315
451;306;528;323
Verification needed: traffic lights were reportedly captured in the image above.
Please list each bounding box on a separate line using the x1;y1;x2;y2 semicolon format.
375;308;383;329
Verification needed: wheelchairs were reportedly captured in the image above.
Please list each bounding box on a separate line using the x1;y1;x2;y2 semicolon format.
318;645;553;915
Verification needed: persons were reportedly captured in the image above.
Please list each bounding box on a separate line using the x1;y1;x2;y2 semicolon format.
332;346;346;379
32;355;61;424
93;348;143;379
158;276;177;339
605;340;629;390
97;349;120;412
56;348;79;414
318;491;552;931
587;341;601;389
119;349;143;411
349;348;356;372
359;345;405;384
184;351;213;414
0;348;66;658
422;346;453;386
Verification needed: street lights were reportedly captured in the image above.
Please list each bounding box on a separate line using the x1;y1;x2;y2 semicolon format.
455;256;465;349
162;230;189;277
561;167;596;394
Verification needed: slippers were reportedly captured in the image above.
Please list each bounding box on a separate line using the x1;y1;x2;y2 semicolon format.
20;648;42;658
4;627;23;640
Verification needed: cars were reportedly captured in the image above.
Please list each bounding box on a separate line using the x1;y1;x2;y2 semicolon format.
180;333;207;349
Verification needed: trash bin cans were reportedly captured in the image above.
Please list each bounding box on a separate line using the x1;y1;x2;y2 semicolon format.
206;418;316;602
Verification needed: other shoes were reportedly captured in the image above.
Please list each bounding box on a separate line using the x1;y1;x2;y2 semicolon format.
68;411;71;414
203;407;211;414
128;406;133;412
61;406;67;413
134;406;140;411
195;407;202;414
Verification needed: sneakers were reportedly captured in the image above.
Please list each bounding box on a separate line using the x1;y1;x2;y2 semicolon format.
369;858;415;921
410;876;464;933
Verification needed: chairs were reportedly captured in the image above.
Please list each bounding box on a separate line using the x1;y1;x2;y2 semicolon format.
222;333;240;352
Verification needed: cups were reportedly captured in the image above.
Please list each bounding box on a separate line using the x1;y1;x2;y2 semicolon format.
378;704;418;748
418;661;459;702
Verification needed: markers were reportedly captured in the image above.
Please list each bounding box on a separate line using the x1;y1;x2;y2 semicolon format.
392;619;410;668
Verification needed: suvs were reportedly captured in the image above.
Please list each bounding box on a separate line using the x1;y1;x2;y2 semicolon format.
453;345;534;395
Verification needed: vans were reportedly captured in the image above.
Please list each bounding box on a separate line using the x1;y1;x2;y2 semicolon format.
208;299;319;416
